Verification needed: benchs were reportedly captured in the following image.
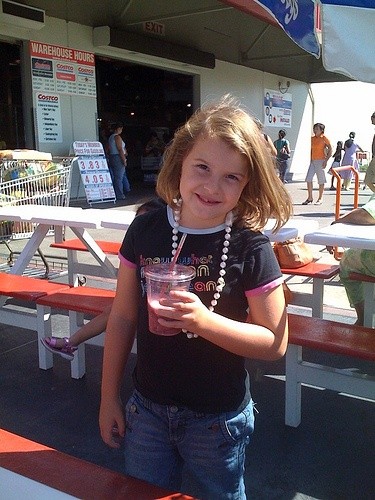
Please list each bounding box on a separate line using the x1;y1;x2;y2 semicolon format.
0;237;375;428
0;429;201;500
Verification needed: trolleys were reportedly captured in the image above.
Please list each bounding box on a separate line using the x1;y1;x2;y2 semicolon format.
353;151;370;189
0;155;80;279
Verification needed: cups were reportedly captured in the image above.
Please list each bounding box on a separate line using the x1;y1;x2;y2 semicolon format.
144;264;195;336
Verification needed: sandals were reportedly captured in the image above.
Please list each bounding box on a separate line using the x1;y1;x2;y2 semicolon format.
39;337;79;360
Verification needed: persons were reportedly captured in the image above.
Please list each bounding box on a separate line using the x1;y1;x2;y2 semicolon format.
327;192;375;325
339;132;364;190
327;141;344;190
104;124;130;193
272;129;290;184
143;130;166;155
40;198;169;361
107;121;131;200
364;111;375;194
302;123;332;206
99;102;289;500
252;117;278;156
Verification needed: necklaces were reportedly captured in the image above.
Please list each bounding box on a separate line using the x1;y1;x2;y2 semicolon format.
171;195;232;338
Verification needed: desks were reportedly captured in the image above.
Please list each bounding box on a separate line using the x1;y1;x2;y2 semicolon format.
0;204;375;308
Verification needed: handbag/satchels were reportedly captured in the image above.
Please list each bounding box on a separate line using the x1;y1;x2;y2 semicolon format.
279;145;290;161
274;237;314;269
